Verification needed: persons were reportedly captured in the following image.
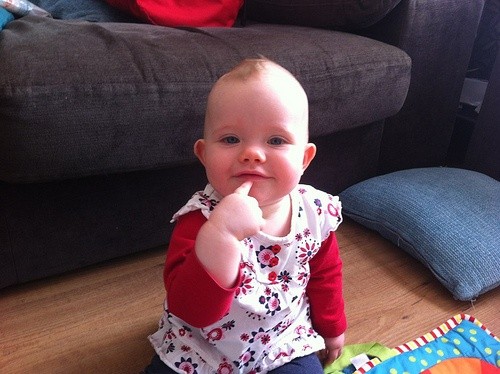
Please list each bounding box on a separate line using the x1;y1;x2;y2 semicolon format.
137;57;346;374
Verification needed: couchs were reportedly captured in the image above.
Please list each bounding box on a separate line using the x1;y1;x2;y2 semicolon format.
0;0;485;300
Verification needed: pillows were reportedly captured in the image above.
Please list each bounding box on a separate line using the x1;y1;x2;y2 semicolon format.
337;167;500;303
